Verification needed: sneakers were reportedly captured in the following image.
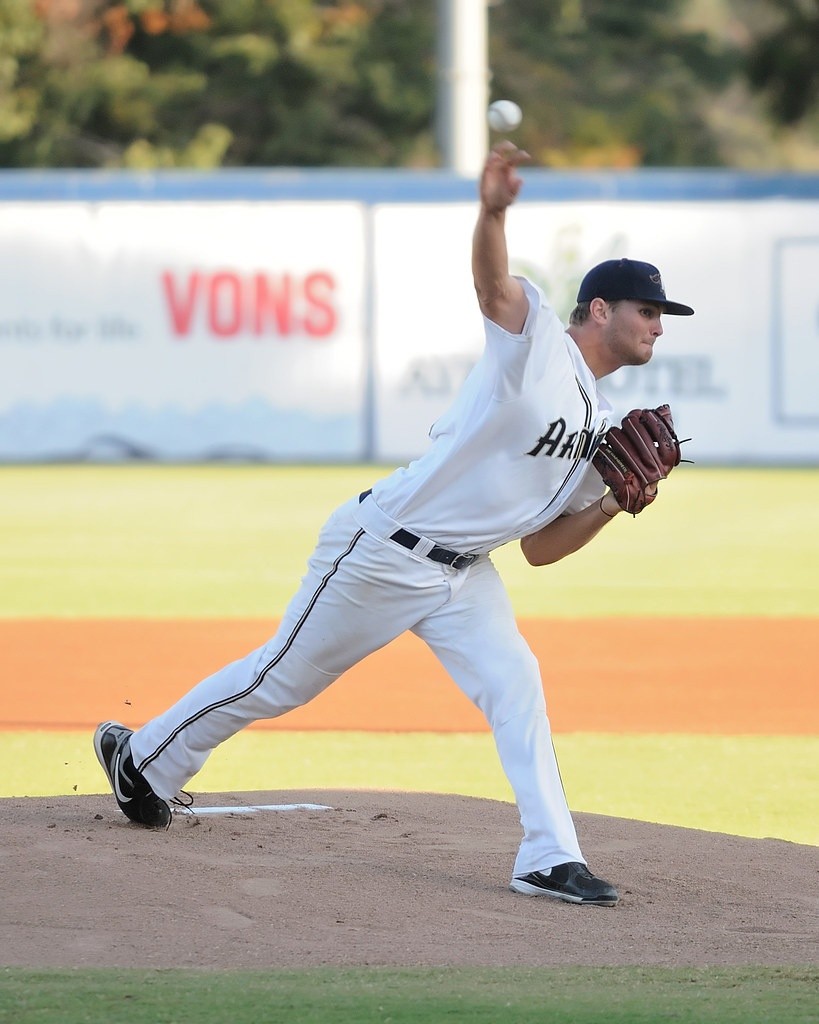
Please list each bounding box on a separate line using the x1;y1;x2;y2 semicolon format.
93;720;194;828
510;862;620;907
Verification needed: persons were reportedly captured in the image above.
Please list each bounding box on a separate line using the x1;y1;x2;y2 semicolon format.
92;139;694;909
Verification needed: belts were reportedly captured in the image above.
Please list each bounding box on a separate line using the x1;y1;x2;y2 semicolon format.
360;488;481;570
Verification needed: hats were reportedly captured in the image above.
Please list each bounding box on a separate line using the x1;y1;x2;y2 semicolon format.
577;257;695;315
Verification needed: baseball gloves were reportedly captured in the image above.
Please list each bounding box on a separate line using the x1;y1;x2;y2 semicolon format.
591;403;694;516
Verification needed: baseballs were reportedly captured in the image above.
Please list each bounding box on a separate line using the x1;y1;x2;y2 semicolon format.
488;100;523;132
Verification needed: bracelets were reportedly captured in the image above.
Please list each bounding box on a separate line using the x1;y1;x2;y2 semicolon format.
600;495;614;518
